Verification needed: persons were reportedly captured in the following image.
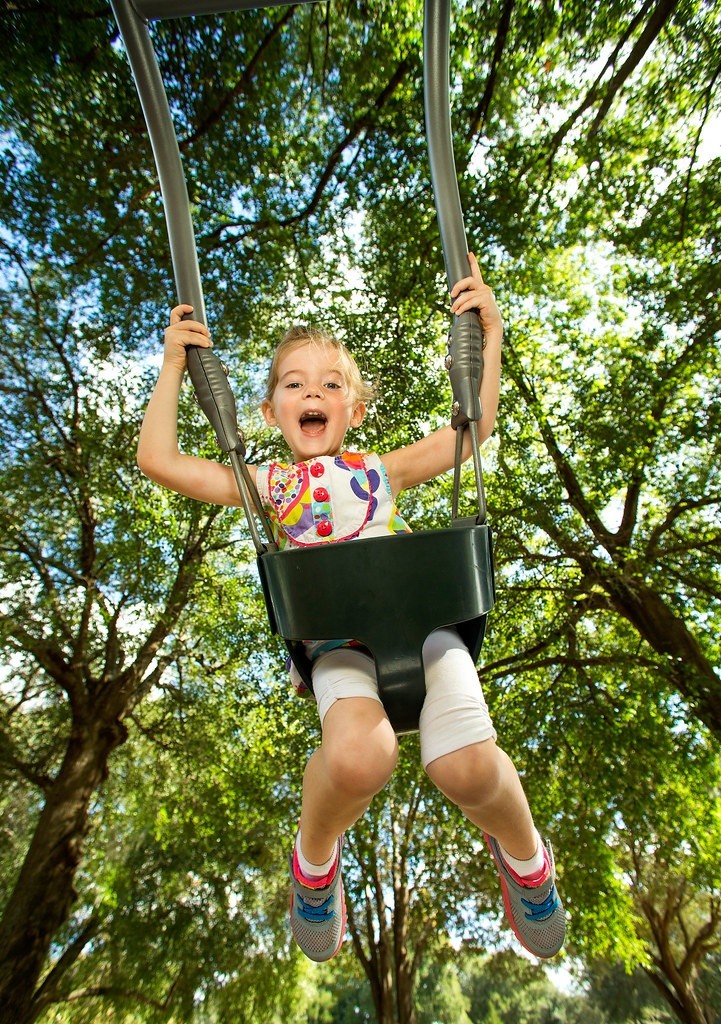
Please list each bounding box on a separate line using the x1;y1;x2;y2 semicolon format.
136;247;569;958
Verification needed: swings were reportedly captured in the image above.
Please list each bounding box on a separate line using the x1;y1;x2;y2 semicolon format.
108;1;496;733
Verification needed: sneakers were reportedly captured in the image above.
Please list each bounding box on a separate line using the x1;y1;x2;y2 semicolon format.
482;827;566;958
288;819;348;962
